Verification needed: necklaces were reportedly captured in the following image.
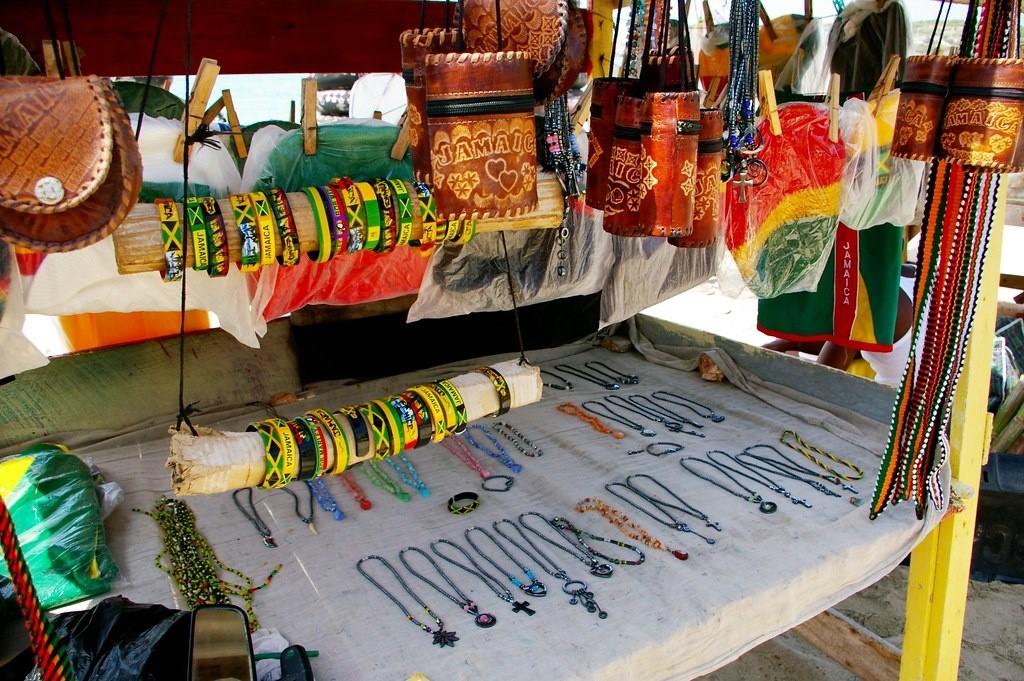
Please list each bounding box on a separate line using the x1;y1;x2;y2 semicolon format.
721;0;769;203
133;359;864;649
544;93;586;277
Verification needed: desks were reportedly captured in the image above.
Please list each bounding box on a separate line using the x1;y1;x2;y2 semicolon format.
42;344;955;680
